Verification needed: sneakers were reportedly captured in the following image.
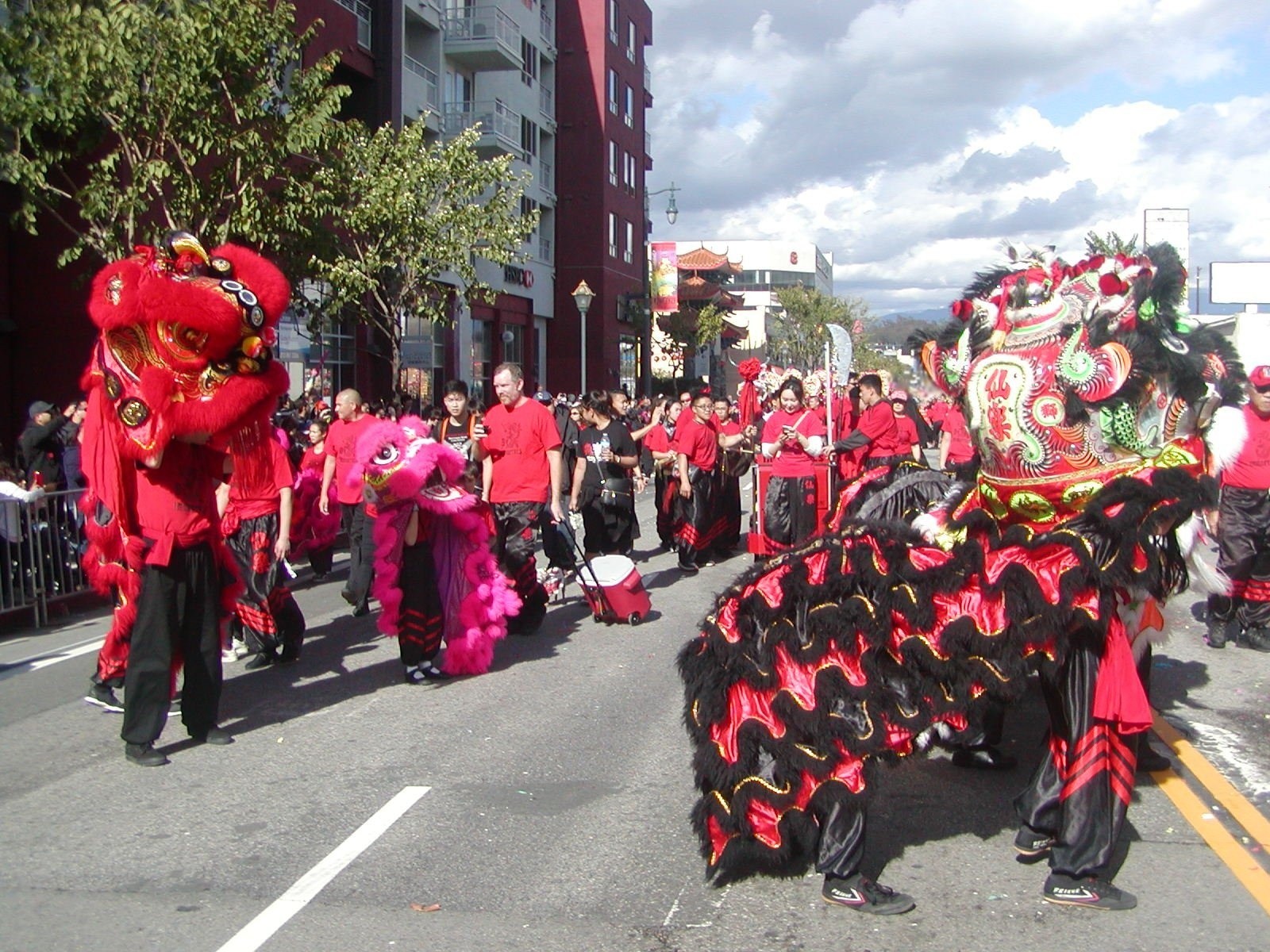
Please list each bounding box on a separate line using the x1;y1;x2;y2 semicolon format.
1042;871;1138;911
123;741;168;766
165;702;182;716
1011;824;1055;856
188;723;234;745
84;685;126;712
820;874;916;915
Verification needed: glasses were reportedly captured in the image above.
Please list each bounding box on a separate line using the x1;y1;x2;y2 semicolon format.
693;402;712;410
679;397;692;405
570;411;578;416
713;406;727;413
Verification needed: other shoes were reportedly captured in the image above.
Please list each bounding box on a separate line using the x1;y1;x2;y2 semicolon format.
1133;746;1171;773
243;650;278;669
661;540;679;552
232;637;248;654
269;643;300;665
404;668;435;688
220;648;238;662
950;744;1014;770
352;598;369;615
1234;625;1270;654
696;558;715;567
419;664;449;681
715;548;733;558
1206;624;1226;648
341;588;359;605
311;572;329;584
678;559;698;571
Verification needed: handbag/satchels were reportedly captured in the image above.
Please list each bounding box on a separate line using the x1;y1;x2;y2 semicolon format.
601;477;632;506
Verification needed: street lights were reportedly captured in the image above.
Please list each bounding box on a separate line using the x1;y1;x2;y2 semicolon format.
570;278;596;397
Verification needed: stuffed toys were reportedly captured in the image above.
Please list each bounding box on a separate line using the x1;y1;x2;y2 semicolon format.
72;231;288;765
347;416;522;683
679;236;1219;915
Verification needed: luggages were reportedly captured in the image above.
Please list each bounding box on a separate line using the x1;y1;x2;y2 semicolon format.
552;514;653;626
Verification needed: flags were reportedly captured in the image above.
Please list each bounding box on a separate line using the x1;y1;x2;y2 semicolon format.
649;242;678;310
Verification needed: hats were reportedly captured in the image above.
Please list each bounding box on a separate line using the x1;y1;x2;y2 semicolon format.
889;391;908;403
28;400;54;420
531;390;552;403
1247;364;1270;389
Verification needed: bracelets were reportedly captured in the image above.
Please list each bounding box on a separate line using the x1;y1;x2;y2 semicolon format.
615;453;622;465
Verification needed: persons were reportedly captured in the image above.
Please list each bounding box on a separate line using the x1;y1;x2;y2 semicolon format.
648;398;682;553
711;397;745;557
564;389;640;565
532;391;581;572
319;388;387;606
1208;363;1270;652
678;393;759;573
2;389;339;673
357;372;982;511
472;362;565;635
